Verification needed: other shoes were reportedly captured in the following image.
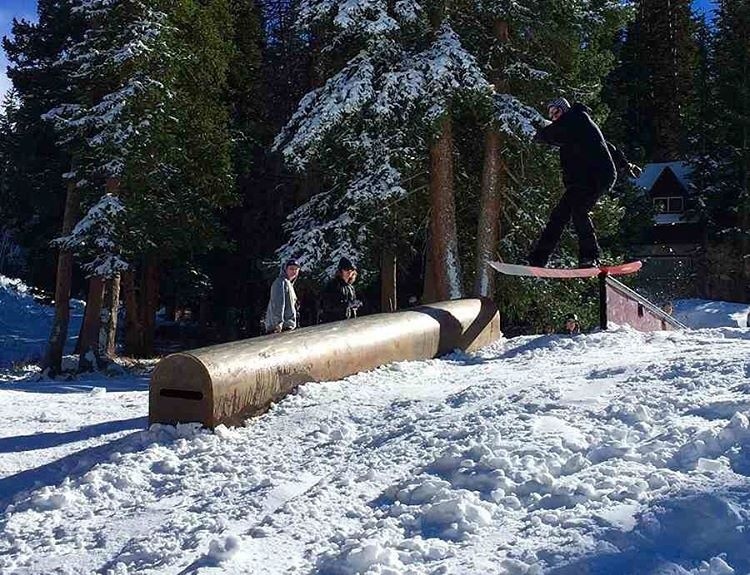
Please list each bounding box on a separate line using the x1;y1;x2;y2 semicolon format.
516;259;601;268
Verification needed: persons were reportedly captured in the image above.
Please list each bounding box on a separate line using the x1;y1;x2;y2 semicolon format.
518;96;644;266
663;300;673;314
266;259;302;335
543;323;554;333
322;258;361;319
556;312;578;334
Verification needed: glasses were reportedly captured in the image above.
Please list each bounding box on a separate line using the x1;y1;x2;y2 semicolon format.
549;109;559;121
343;266;354;271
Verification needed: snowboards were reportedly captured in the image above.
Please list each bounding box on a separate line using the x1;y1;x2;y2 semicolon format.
483;259;644;279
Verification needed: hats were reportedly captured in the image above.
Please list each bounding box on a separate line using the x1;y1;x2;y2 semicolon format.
338;257;352;269
283;257;301;271
546;97;571;114
561;314;578;323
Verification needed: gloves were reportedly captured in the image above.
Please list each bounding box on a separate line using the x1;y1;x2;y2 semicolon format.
625;162;643;179
528;116;546;130
